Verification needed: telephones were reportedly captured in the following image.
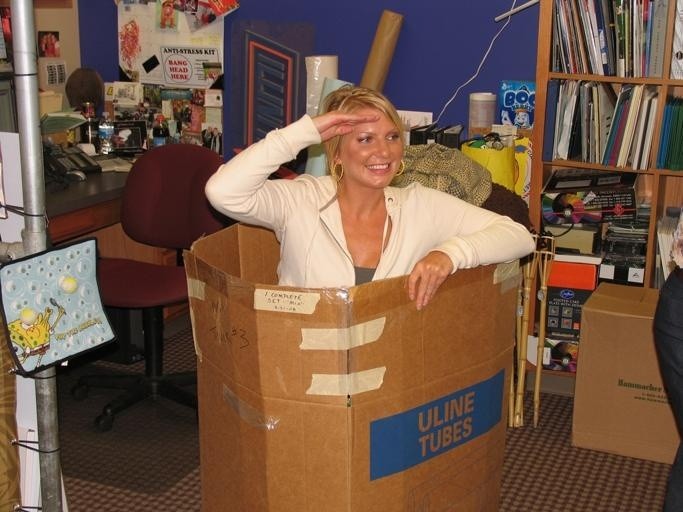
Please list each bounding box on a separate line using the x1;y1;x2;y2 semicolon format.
42;143;79;183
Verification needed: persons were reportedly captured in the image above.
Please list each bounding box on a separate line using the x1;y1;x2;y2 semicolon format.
203;85;536;310
653;207;683;512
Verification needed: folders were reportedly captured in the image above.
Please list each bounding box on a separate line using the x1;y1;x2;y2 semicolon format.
409;122;466;152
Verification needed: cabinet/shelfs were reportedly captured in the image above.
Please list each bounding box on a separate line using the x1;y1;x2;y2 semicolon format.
519;0;682;401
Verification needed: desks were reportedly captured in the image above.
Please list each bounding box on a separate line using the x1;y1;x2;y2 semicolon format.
44;163;189;325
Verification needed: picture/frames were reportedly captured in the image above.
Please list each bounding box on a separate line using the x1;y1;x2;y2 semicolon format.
99;119;148;154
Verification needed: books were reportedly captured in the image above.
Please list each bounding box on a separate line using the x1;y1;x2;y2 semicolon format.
542;0;683;171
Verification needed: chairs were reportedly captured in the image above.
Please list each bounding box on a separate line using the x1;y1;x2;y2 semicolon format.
69;142;228;433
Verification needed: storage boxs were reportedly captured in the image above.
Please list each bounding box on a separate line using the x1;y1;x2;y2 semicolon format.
569;281;680;468
548;222;598;258
542;255;598;293
538;287;596;337
179;223;521;512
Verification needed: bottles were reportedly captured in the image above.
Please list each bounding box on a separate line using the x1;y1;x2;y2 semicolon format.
78;102;115;155
468;92;497;137
152;115;169;147
